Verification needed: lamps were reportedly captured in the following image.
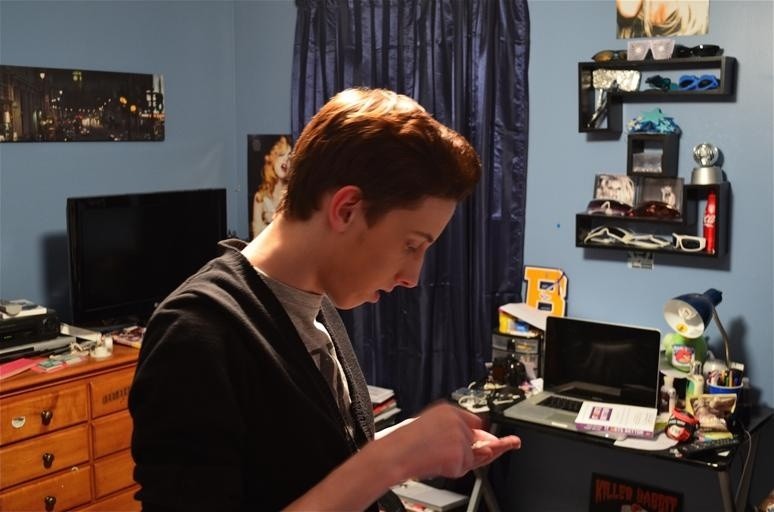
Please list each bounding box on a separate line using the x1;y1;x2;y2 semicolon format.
665;287;739;387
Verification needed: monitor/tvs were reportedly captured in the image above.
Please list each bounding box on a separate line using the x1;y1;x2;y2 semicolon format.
65;188;227;327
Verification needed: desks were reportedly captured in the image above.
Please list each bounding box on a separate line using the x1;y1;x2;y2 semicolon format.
450;385;774;511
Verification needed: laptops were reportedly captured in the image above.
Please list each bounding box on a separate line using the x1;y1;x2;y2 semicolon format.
503;316;661;441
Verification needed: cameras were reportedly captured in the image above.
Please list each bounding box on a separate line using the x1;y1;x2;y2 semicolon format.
491;352;527;388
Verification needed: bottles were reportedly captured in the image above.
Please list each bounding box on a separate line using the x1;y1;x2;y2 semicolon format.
491;338;526;385
685;362;705;414
658;376;677;413
703;191;718;252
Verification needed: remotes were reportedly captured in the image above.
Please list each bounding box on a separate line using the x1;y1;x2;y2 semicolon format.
678;438;741;455
0;299;22;316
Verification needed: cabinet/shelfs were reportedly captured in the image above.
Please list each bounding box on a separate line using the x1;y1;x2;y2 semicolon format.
0;337;142;510
572;55;741;271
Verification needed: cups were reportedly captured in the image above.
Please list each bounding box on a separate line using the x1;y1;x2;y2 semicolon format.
706;377;743;426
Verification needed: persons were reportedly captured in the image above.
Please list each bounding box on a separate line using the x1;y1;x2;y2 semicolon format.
251;135;292;237
126;85;524;512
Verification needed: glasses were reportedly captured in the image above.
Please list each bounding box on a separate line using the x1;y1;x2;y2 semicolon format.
458;394;487;409
586;81;618;126
591;39;721;90
586;199;680;219
584;226;706;252
628;107;677;133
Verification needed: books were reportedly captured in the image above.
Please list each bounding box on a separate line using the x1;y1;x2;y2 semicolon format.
575;398;659;440
0;299;147;382
367;385;470;511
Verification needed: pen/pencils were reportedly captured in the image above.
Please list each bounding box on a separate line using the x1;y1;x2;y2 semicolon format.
707;369;742;387
